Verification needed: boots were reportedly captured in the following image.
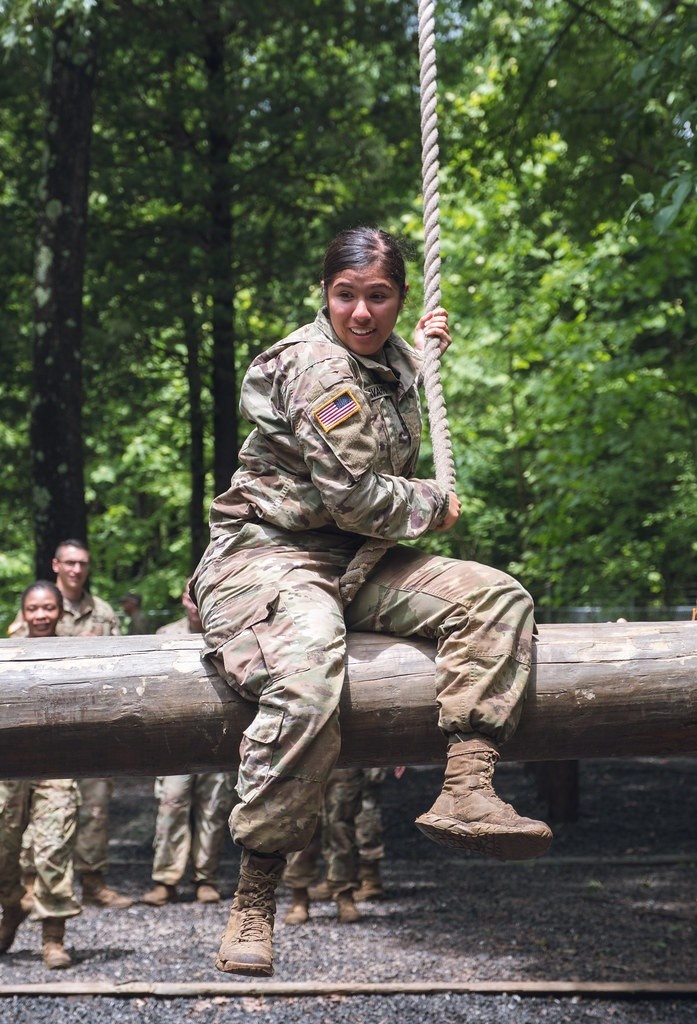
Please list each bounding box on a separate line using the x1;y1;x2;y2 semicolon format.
42;919;71;969
336;891;362;923
0;900;31;952
285;896;309;925
79;872;132;908
215;850;287;976
415;733;553;862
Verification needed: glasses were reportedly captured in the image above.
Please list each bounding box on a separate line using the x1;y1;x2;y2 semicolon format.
60;560;87;568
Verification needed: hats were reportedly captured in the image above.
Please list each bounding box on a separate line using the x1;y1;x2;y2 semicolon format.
119;594;141;602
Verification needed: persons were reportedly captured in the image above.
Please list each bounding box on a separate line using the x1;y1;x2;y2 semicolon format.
195;226;555;974
139;573;233;907
5;541;137;910
0;580;87;971
118;588;150;635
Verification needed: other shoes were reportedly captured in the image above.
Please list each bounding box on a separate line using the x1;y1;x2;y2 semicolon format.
196;884;220;903
308;877;381;901
143;885;177;905
